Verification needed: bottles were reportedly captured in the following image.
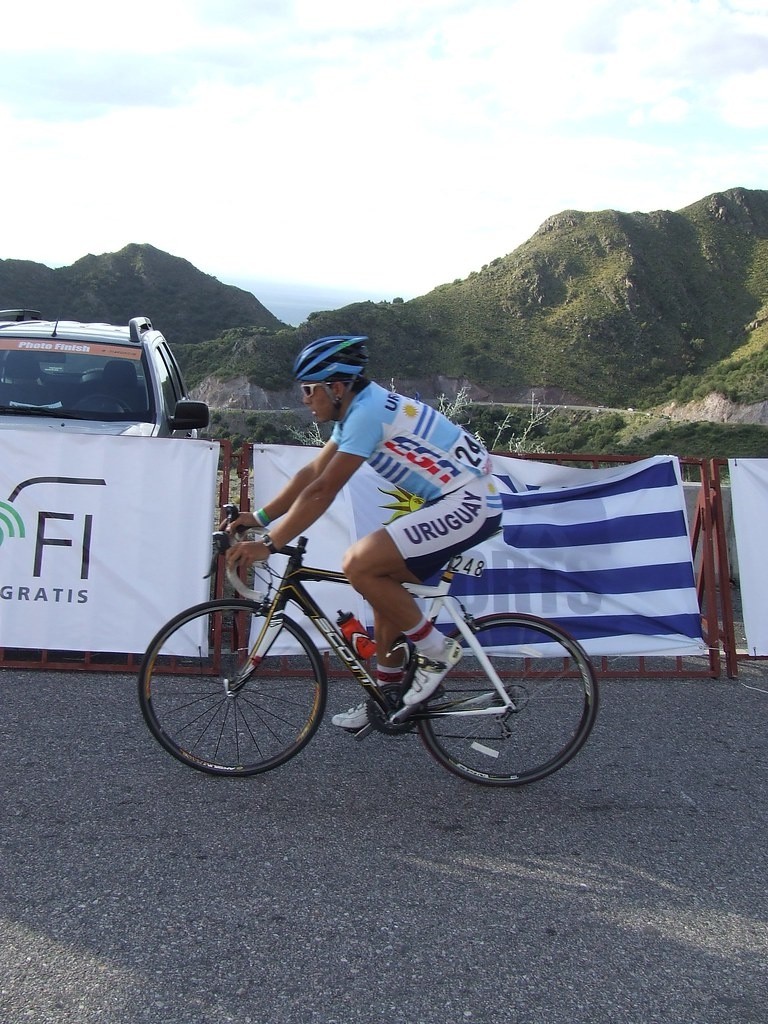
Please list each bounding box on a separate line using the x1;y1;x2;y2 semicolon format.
336;609;377;660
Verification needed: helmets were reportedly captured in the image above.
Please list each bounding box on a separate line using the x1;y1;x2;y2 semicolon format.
292;336;368;380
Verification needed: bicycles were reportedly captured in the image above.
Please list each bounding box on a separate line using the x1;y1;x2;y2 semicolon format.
138;504;600;787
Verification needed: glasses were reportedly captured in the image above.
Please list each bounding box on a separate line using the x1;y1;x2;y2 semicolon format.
301;383;331;398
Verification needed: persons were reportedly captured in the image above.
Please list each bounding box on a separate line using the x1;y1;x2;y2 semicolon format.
218;335;504;729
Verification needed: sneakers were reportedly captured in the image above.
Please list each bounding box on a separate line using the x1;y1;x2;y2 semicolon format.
332;703;369;728
402;639;464;704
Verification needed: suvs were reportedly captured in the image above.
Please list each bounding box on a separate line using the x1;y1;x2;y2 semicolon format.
0;309;210;440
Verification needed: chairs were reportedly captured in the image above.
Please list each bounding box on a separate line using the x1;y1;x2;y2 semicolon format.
0;349;145;413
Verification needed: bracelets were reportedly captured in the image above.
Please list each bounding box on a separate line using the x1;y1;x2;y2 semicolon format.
261;533;277;554
252;508;271;527
